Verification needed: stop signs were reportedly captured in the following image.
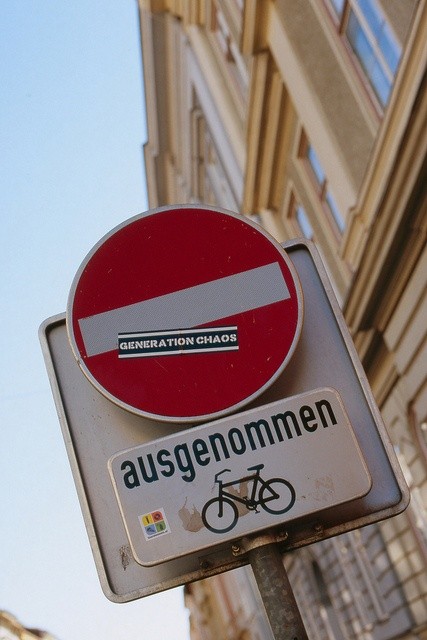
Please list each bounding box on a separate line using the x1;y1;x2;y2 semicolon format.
65;203;305;424
